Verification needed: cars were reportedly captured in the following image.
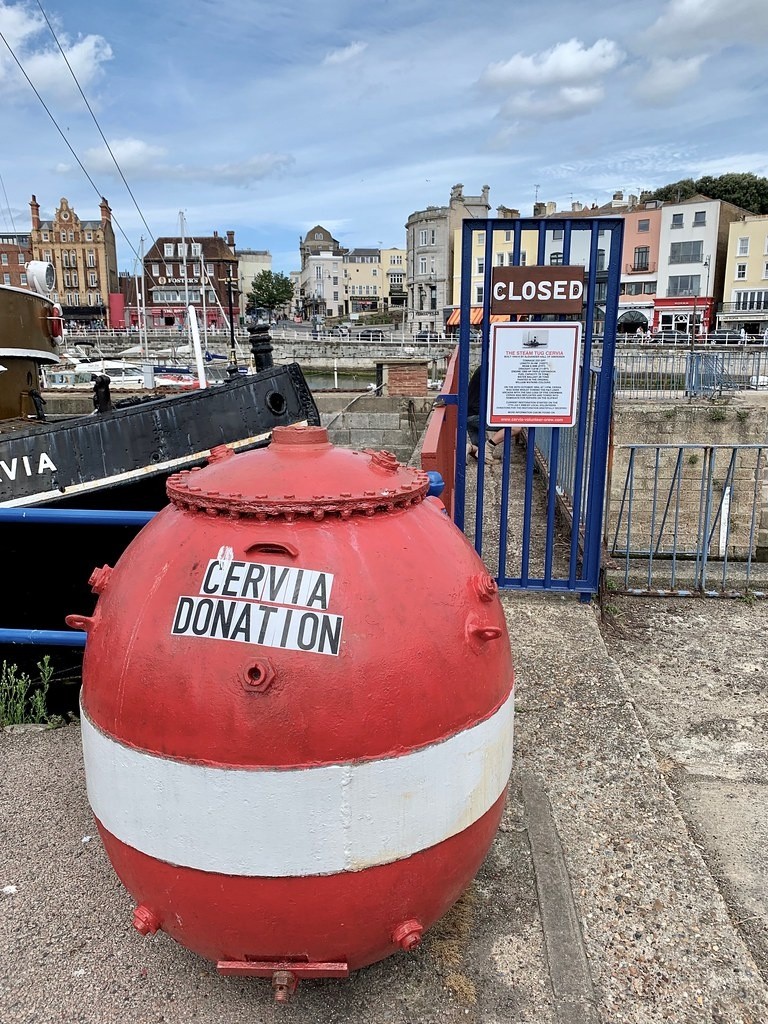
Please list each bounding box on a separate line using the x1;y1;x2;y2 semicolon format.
356;329;389;339
327;325;351;336
413;330;446;341
697;328;748;343
649;329;696;342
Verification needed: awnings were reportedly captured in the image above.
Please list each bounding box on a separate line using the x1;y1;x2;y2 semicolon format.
446;308;521;326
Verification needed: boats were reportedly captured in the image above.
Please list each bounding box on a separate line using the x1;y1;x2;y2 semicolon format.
37;213;254;393
0;261;323;699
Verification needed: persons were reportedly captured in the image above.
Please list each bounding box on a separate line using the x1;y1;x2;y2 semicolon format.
467;365;522;465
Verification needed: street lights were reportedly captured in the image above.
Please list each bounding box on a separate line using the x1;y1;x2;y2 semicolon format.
702;254;711;335
